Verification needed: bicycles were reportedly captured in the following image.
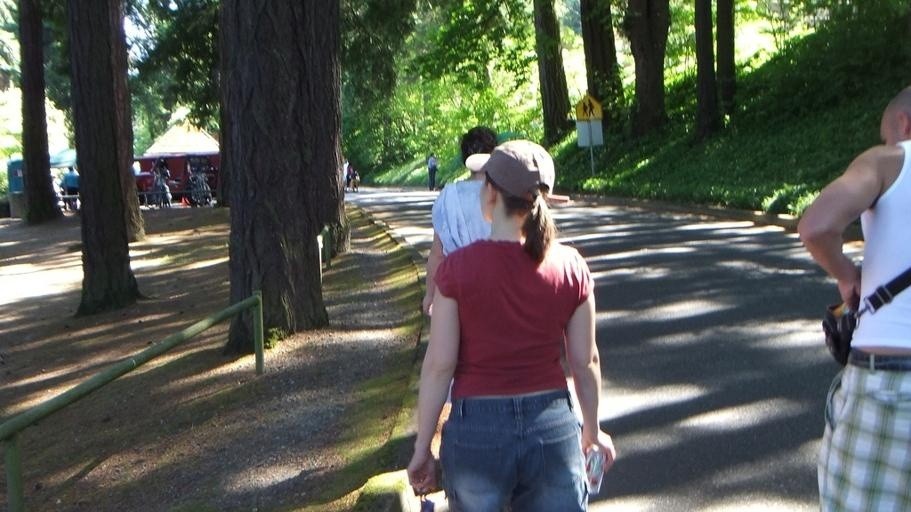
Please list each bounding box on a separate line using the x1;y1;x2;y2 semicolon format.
153;172;171;208
184;174;213;207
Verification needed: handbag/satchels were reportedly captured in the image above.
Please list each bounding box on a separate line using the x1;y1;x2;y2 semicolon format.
822;302;857;366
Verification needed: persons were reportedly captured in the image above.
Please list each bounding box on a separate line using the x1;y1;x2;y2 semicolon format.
428;152;439;191
404;137;619;511
151;157;173;207
347;162;354;190
798;87;910;512
422;127;501;314
52;166;80;210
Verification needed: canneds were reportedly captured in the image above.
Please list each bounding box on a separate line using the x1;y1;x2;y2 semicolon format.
586;441;609;497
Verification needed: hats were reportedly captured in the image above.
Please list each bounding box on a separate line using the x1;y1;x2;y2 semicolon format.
464;139;556;202
